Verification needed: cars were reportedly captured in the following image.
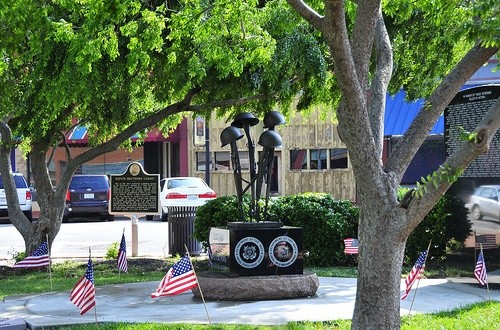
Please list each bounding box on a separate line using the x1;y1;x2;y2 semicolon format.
146;178;217;222
61;175;114;223
0;172;37;223
465;184;500;223
